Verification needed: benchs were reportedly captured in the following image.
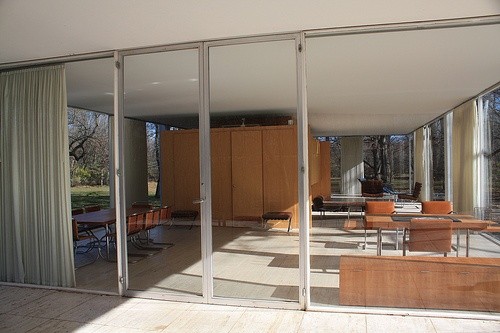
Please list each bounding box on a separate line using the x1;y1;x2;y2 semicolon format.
261;212;293;233
170;210;198;230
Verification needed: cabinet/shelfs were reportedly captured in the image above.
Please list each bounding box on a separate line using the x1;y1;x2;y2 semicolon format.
159;126;311;227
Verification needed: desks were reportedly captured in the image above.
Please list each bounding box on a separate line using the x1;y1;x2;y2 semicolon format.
364;214;489;257
74;210;167;263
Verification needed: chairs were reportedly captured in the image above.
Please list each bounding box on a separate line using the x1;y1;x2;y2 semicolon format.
403;219;452;256
72;207;172;273
362;180;384;197
398;182;421;209
420;201;456;214
364;201;398;215
